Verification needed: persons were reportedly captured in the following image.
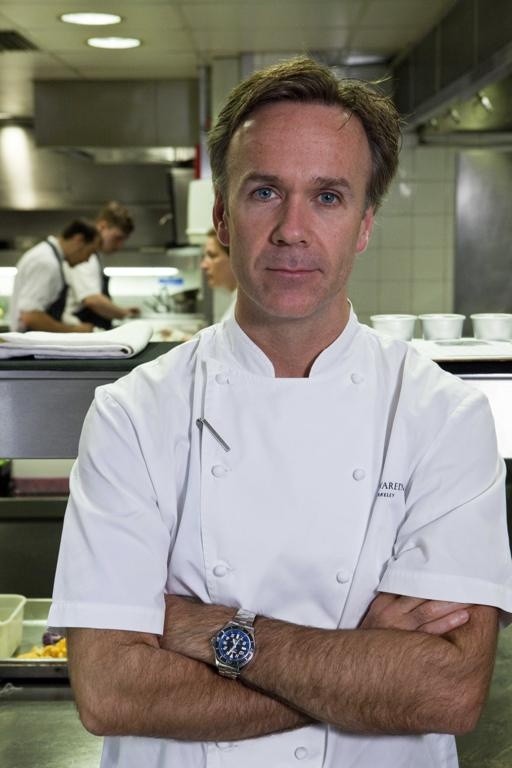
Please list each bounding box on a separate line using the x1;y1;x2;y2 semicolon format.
47;60;512;768
61;201;139;330
8;219;101;333
200;229;236;291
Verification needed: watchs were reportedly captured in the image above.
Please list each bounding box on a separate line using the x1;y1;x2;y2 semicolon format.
210;608;258;679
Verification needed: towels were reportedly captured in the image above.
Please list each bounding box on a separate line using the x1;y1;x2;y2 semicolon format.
0;316;158;365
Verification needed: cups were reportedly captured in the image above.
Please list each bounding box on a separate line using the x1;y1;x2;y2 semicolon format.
367;312;418;341
418;312;466;344
469;311;512;342
